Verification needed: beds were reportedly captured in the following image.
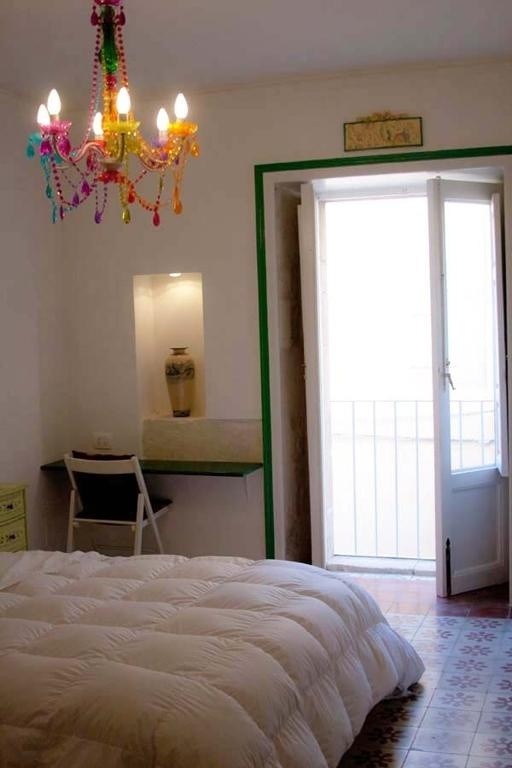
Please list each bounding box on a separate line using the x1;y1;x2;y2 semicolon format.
0;550;398;767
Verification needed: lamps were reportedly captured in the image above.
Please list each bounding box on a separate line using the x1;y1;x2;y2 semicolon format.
22;1;203;232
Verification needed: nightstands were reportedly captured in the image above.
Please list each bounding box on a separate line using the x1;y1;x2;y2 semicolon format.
1;483;31;551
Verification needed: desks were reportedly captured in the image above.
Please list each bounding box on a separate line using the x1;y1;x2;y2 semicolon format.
40;451;264;504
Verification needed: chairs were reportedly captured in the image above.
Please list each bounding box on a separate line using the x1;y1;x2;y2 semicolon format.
58;453;174;555
